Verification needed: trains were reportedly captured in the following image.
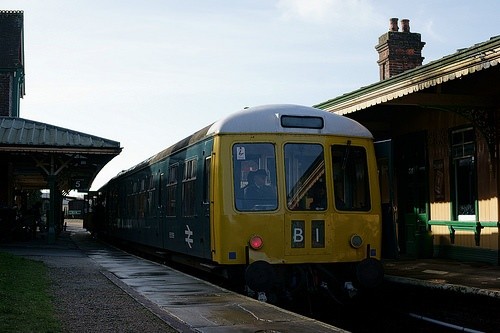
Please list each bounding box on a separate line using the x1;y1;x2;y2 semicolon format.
81;107;382;301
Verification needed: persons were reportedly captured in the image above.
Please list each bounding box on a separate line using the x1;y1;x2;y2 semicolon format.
243;169;274;200
30;201;45;233
240;171;257;200
